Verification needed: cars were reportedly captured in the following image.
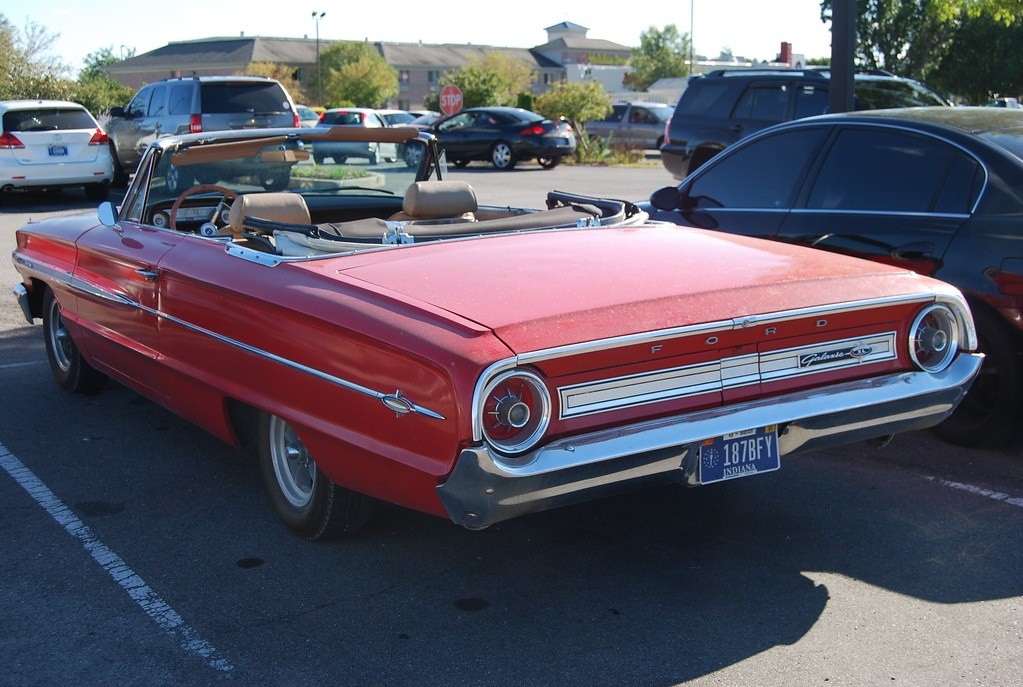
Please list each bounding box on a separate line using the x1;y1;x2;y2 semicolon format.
394;106;577;171
293;103;442;167
630;106;1022;448
0;101;114;208
11;126;987;548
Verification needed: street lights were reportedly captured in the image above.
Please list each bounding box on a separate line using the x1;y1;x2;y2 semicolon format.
311;11;326;108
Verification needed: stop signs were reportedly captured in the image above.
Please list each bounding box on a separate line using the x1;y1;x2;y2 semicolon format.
439;86;464;117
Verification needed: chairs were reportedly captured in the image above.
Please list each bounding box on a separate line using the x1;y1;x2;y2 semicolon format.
7;117;20;131
387;182;476;222
631;114;640;122
218;194;310;242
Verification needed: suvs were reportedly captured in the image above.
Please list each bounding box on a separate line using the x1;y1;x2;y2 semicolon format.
102;75;305;200
661;66;954;180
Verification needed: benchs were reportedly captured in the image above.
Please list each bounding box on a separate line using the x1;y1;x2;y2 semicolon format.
242;190;648;244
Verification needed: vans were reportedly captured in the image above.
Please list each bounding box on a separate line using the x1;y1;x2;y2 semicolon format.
583;101;675;151
